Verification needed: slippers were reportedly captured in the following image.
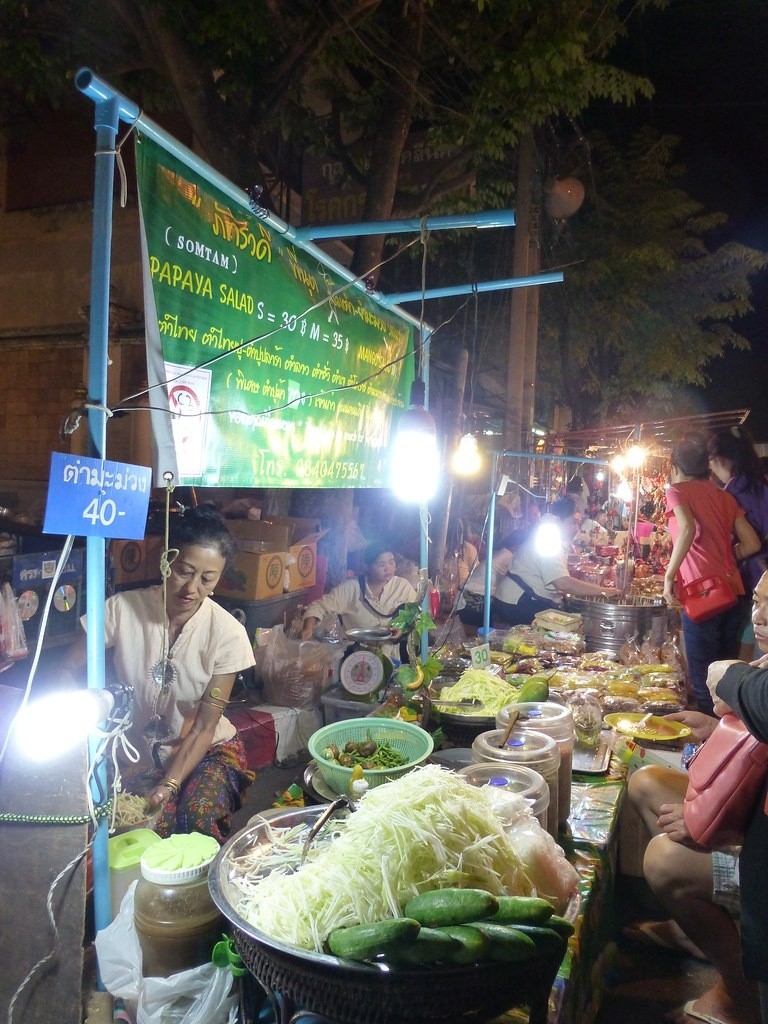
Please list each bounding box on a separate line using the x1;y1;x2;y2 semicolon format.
611;917;720;960
665;998;727;1024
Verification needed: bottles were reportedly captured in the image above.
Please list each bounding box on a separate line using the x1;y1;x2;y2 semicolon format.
134;835;225;982
680;743;695;768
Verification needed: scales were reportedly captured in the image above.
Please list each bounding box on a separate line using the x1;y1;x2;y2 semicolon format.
338;627;395;704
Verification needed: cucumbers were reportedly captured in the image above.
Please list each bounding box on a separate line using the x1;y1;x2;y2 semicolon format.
327;888;575;965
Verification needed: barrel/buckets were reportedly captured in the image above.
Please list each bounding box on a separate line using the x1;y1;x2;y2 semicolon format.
563;595;668;657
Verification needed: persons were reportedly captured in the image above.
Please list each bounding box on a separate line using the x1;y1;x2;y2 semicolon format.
454;493;624;628
298;543;421;664
625;567;768;1024
53;503;258;848
662;425;768;720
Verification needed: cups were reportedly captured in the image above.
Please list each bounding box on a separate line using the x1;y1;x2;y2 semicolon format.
474;730;559;841
495;700;573;822
452;764;549;833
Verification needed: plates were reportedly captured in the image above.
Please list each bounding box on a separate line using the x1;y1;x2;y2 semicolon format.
602;713;693;743
429;748;474;770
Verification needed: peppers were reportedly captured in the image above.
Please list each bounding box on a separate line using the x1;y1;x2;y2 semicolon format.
407;666;424;688
430;588;440;619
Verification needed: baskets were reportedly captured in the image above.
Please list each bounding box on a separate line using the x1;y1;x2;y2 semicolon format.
308;718;434;796
207;800;580;1024
108;797;164;838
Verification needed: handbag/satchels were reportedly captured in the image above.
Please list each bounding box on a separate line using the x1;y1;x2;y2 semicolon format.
676;575;738;623
260;624;355;707
684;712;768;847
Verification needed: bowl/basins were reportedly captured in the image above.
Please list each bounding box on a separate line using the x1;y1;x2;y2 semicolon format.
207;804;578;988
113;798;163;837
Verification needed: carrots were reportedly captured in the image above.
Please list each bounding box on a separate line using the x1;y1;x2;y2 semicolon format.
434;669;521;717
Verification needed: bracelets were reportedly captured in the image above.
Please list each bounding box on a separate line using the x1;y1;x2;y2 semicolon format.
202;689;229;711
159;774;183;794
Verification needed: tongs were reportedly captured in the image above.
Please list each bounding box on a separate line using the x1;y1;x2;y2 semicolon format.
302;793;358;868
423;697;481;709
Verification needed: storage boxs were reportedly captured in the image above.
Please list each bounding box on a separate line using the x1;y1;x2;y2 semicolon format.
215;588;311;641
321;687;380;724
147;534;165;582
534;609;582;632
214;520;291;602
264;514;332;592
111;538;145;585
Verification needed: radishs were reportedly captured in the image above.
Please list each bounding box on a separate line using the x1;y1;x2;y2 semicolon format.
224;761;560;954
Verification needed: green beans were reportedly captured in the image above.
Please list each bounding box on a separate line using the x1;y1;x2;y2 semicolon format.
341;728;410;770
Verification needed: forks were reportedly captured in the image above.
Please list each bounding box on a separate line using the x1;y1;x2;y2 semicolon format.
635;713;653;729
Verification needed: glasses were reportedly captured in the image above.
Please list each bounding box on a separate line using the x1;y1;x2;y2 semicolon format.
707;453;718;469
